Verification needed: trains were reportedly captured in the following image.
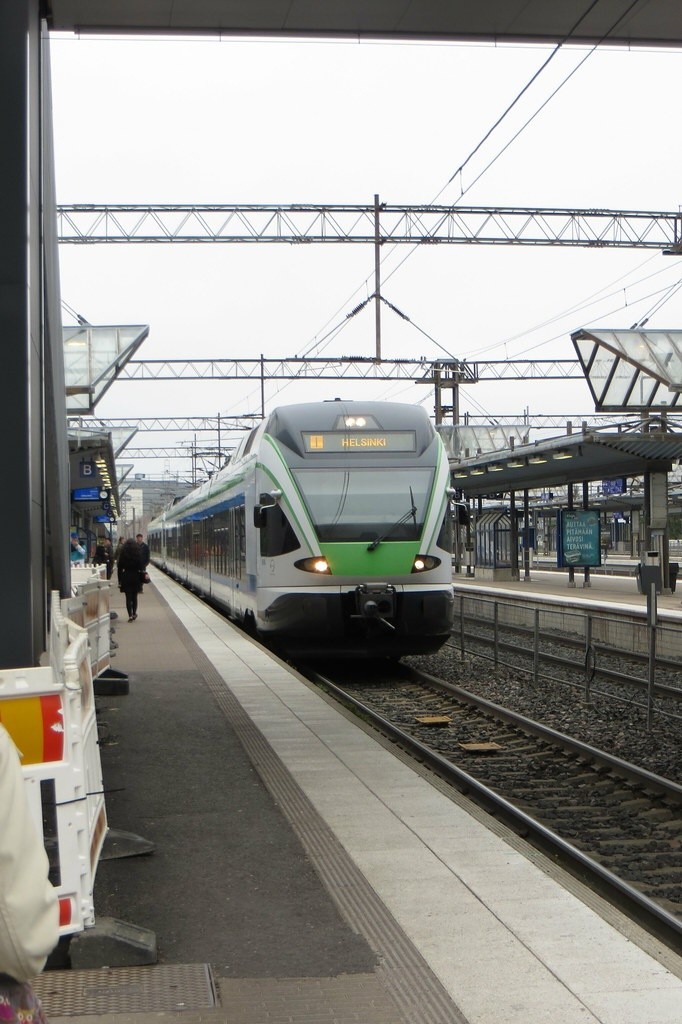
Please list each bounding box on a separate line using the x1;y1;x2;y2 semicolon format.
147;398;470;671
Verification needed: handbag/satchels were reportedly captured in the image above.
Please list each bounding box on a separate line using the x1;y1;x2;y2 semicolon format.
139;566;151;584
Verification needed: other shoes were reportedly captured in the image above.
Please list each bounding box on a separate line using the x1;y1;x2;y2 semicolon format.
133;614;137;619
128;619;133;622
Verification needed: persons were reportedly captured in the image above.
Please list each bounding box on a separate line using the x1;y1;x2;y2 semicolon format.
117;540;144;624
71;532;87;568
91;534;151;594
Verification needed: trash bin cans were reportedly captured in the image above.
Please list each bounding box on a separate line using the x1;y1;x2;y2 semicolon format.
634;550;679;595
